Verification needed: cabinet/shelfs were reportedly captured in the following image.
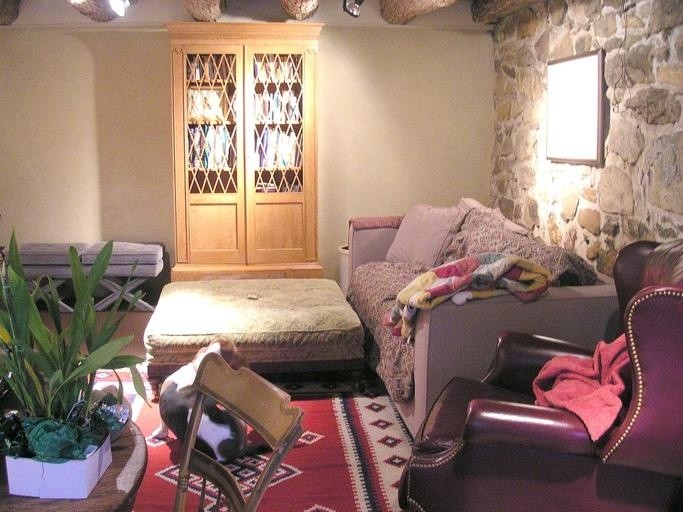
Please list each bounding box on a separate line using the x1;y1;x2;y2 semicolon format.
165;20;327;264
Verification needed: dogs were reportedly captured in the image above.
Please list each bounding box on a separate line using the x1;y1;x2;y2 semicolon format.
152;338;273;464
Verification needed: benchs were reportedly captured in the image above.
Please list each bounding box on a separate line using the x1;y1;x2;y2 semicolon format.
0;240;165;310
143;278;365;400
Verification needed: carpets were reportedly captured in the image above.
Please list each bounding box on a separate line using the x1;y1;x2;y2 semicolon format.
72;360;415;512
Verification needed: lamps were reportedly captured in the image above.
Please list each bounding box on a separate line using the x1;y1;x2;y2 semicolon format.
110;0;137;18
343;0;364;17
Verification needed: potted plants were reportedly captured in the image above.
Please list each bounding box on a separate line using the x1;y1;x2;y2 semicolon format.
0;227;146;501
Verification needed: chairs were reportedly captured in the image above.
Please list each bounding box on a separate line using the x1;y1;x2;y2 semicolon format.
398;239;683;512
174;353;307;512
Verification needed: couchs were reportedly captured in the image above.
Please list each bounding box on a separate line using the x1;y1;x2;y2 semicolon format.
348;217;622;446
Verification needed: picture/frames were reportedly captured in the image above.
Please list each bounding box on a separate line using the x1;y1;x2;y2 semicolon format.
543;47;605;166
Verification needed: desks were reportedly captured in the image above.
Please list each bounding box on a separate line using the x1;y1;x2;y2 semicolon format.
0;418;147;512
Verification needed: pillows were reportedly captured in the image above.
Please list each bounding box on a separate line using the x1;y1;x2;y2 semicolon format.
386;197;599;288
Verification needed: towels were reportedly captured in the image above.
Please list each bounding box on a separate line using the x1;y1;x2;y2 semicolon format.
530;325;631;444
